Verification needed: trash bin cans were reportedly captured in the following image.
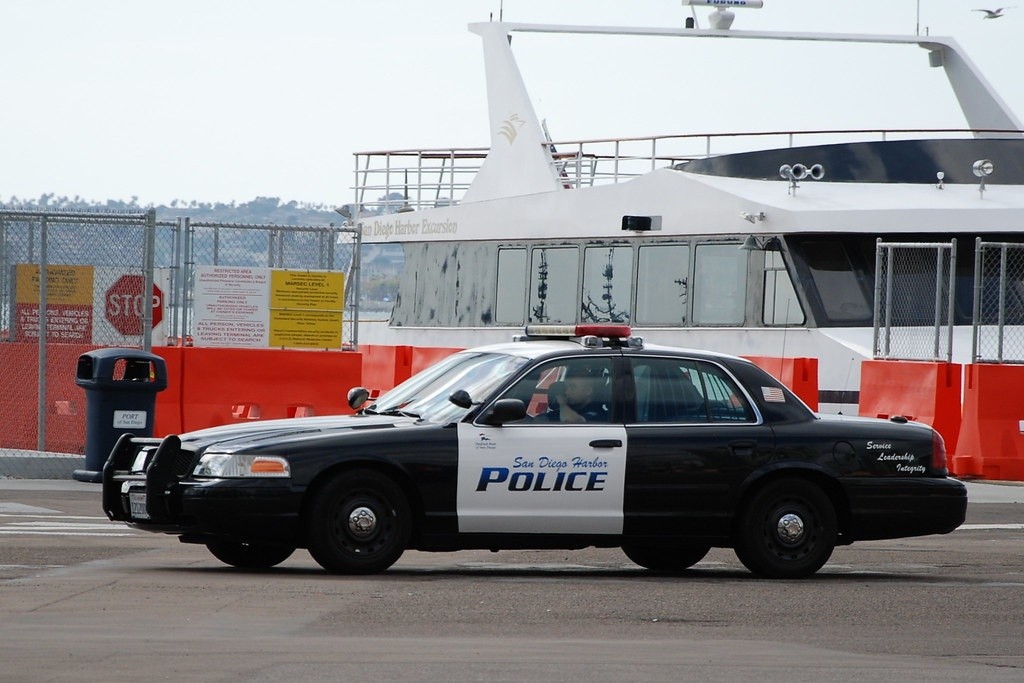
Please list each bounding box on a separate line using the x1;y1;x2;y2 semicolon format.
74;348;168;482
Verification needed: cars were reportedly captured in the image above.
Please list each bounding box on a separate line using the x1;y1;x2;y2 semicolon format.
102;324;968;577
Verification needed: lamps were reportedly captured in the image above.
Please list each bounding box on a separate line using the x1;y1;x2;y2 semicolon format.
972;159;994;190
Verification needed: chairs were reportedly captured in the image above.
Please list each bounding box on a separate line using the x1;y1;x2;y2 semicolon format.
547;381;564;414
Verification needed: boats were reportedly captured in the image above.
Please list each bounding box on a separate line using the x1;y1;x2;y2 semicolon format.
337;0;1024;418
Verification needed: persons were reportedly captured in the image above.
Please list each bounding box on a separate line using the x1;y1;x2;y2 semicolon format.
513;368;606;424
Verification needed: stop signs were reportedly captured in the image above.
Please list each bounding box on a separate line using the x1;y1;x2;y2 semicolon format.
104;273;166;335
